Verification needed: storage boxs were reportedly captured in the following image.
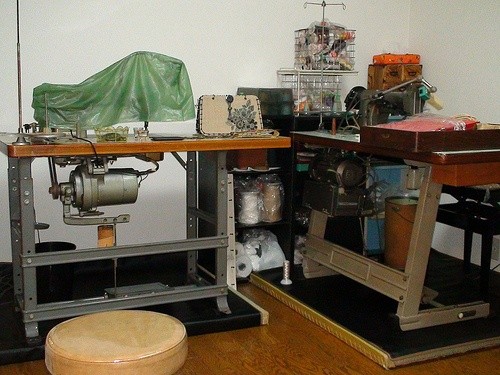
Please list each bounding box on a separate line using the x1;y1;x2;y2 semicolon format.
366;166;425;215
294;28;359;73
305;181;366;216
363;217;386;256
360;123;500;152
276;69;343;114
366;63;423;89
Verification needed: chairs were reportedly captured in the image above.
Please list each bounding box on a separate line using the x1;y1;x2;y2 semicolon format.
435;183;500;271
33;207;77;254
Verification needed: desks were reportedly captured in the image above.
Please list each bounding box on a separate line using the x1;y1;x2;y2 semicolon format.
0;128;291;366
289;130;500;330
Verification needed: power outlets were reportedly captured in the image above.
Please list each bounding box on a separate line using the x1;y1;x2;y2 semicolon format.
491;237;500;262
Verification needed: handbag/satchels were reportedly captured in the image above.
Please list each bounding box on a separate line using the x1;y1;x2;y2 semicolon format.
192;92;280;138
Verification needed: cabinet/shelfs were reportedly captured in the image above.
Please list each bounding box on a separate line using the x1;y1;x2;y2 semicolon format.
198;149;294;280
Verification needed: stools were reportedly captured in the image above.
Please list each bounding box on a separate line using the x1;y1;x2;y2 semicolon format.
45;309;189;375
